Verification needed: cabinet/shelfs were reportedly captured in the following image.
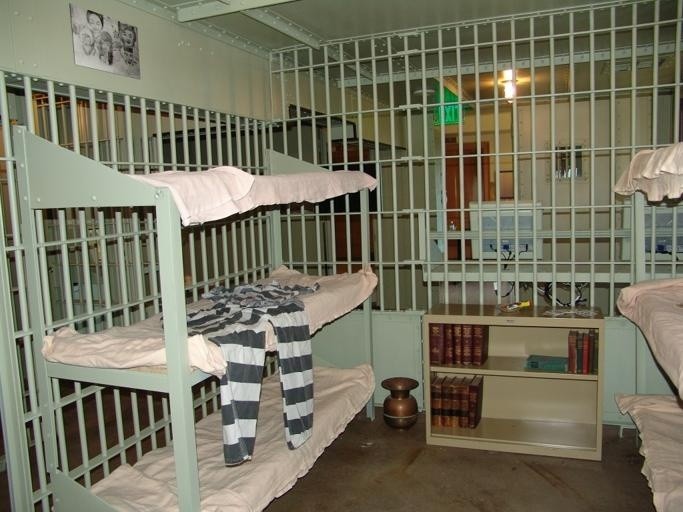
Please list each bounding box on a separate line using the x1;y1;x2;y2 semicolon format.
421;309;605;461
325;137;406;308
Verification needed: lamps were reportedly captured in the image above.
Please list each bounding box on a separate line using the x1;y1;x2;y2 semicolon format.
501;69;518;104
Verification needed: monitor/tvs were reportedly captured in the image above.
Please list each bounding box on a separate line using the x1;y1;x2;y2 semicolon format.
622;191;683;262
469;202;542;260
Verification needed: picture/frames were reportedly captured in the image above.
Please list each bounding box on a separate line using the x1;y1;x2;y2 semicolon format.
70;3;140;78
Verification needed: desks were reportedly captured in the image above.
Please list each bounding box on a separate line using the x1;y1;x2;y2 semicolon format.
403;210;682;304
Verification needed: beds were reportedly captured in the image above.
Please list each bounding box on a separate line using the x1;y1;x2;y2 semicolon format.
15;128;367;211
52;362;374;512
613;141;682;512
43;268;374;396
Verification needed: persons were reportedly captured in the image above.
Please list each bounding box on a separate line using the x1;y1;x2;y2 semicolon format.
118;27;138;67
95;33;114;64
78;28;96;57
82;10;103;34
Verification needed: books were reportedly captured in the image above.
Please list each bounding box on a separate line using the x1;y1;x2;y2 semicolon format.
450;377;465;428
469;374;483;427
453;324;462;364
442;376;456;427
583;333;588;373
523;353;568;373
430;323;444;366
588;328;594;374
567;329;578;374
443;324;454;366
460;376;473;428
594;332;598;375
577;332;583;373
473;325;489;365
431;376;446;425
461;323;473;366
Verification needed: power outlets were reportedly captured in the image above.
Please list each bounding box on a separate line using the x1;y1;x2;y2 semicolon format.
502;268;513;281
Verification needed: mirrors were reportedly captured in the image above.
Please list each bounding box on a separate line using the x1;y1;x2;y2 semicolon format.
545;143;589;183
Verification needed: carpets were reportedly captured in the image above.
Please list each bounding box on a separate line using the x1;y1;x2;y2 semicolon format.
263;406;656;512
